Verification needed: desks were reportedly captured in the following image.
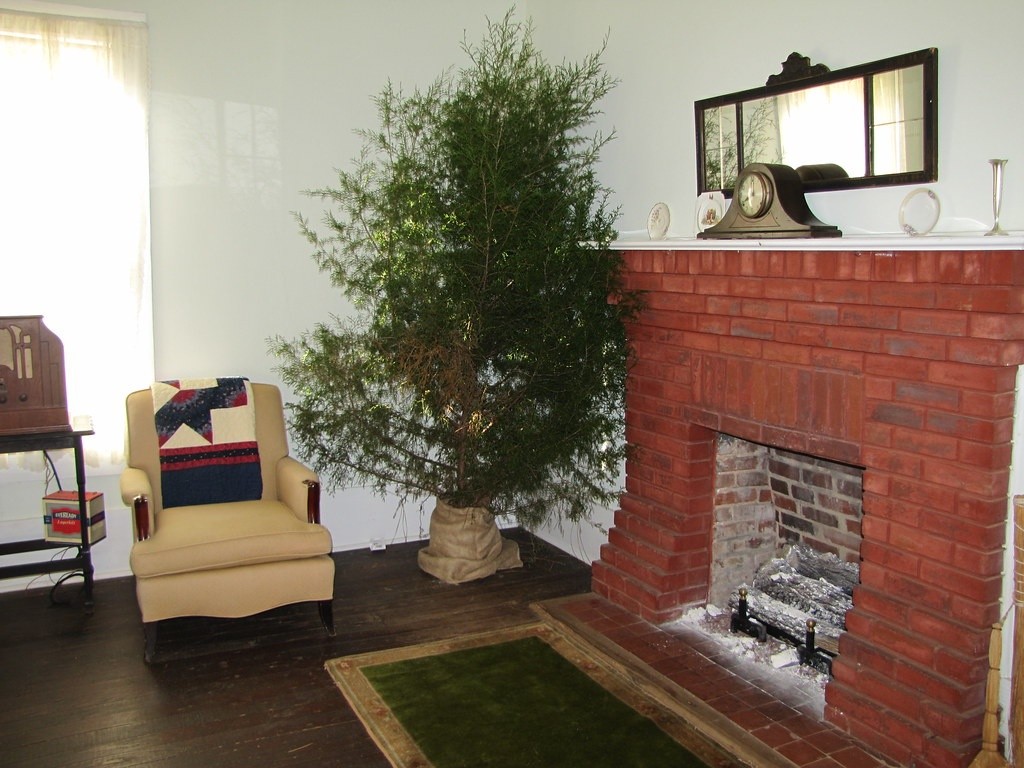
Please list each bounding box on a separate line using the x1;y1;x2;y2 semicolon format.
0;416;96;617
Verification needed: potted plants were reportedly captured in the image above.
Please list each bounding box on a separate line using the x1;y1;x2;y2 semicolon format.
267;6;650;585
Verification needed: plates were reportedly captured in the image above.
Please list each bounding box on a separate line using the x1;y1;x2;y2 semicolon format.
695;191;726;239
898;188;940;237
647;202;670;240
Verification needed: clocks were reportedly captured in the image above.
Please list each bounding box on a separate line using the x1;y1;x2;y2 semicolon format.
695;163;841;241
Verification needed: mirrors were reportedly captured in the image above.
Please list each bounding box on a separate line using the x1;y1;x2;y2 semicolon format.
692;48;939;198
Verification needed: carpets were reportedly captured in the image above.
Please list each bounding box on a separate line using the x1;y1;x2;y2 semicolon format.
324;620;752;768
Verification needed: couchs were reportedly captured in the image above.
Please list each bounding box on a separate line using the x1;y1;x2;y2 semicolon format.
120;375;341;665
